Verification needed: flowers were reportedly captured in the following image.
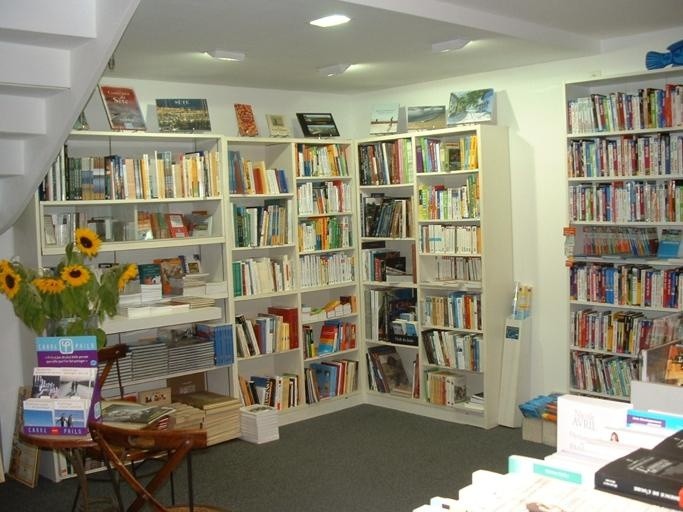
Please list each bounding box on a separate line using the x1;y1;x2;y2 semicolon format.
0;228;138;351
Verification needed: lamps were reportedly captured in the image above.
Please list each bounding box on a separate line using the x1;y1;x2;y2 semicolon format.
319;64;350;77
433;39;469;53
203;48;245;62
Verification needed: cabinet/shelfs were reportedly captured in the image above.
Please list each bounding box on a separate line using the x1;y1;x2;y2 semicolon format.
564;66;683;401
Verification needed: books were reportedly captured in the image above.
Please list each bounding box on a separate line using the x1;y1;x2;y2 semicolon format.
39;82;683;511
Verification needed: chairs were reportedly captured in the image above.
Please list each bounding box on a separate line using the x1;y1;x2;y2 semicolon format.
68;344;207;512
